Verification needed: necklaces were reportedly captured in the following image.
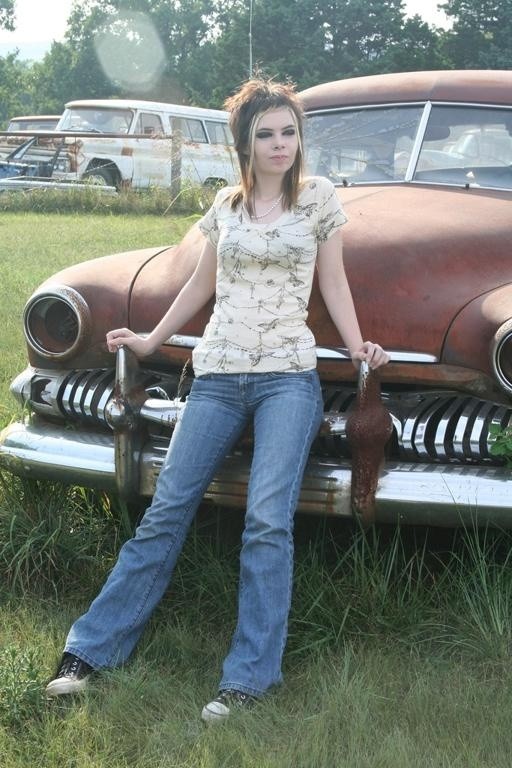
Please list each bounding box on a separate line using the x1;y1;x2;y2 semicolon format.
241;190;285;220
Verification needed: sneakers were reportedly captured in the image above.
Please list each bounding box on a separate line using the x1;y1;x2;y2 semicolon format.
201;689;258;725
45;653;95;697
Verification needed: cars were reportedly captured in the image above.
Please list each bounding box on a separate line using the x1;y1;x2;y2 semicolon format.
6;116;63;176
0;69;511;532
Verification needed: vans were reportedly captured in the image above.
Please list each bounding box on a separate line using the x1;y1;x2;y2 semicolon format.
50;99;243;192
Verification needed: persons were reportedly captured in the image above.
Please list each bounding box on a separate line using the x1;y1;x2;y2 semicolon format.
45;80;391;725
